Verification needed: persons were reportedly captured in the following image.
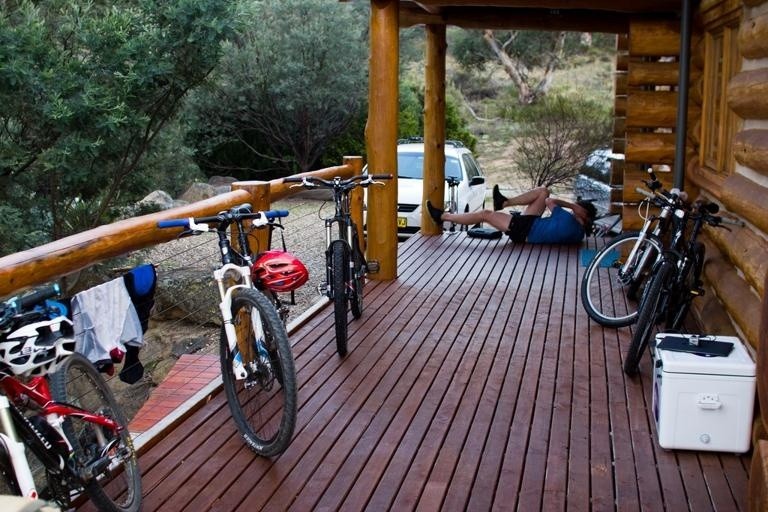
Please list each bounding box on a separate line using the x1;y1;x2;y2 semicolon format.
580;32;592;56
425;184;598;245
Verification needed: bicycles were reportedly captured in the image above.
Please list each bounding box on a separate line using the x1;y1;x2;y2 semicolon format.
580;159;746;376
281;173;394;358
0;276;142;512
156;202;296;459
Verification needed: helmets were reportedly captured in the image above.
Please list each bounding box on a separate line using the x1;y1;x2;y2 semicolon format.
0;311;77;379
250;249;308;293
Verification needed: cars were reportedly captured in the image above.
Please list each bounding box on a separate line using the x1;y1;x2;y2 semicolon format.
574;147;625;234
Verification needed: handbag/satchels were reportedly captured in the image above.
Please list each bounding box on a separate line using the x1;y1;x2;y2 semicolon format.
467;228;502;239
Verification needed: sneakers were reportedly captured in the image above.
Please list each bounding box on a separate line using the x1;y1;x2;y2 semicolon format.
425;200;443;225
493;185;508;211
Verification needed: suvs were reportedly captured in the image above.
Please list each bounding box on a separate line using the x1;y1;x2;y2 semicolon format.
361;138;485;239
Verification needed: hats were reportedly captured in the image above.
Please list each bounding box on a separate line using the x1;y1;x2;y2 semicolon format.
578;200;598;237
